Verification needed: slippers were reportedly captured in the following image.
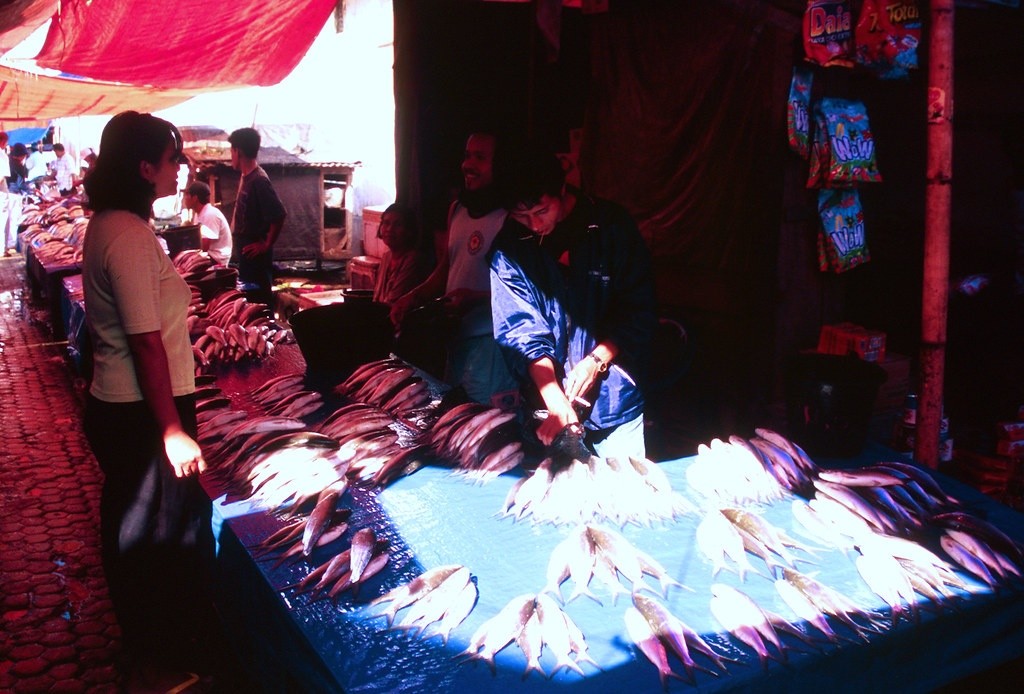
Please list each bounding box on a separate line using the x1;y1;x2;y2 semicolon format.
122;667;217;694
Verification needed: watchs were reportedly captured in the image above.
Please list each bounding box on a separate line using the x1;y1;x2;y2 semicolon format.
589;352;607;373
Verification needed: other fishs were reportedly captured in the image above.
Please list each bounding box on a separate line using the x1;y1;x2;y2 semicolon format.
173;248;297;376
194;357;1024;694
18;198;90;269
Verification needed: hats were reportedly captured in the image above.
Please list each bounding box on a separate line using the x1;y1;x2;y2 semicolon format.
180;181;211;196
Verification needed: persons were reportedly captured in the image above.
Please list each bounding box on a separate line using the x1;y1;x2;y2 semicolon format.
180;181;233;267
370;205;426;305
488;149;654;462
80;109;211;694
387;118;524;411
0;131;98;258
227;127;286;316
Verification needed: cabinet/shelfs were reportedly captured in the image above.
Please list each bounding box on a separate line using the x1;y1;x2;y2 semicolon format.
188;160;364;280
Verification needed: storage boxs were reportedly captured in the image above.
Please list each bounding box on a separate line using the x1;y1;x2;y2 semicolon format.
800;322;910;452
363;205;392;259
350;256;380;290
